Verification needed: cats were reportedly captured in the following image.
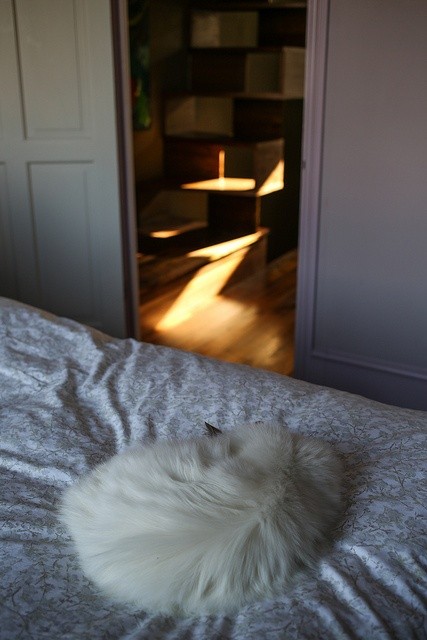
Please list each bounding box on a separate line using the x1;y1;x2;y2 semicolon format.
54;421;346;619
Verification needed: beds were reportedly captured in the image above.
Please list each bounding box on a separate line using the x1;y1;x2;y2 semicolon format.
1;296;427;640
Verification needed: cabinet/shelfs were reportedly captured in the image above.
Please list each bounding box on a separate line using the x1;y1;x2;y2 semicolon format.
135;0;307;294
